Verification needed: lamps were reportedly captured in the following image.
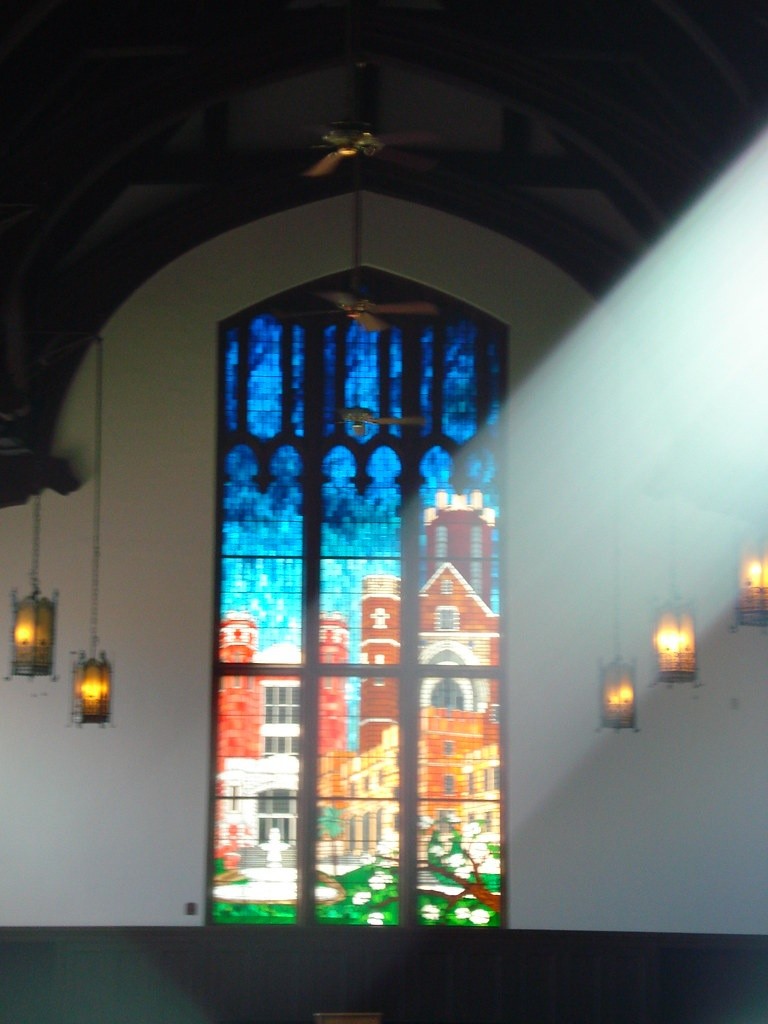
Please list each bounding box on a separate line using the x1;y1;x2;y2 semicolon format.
733;551;768;628
599;660;637;729
69;327;113;727
8;492;62;677
652;603;698;682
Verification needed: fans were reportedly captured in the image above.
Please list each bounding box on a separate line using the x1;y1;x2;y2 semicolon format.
275;187;438;335
314;406;426;438
288;42;449;181
